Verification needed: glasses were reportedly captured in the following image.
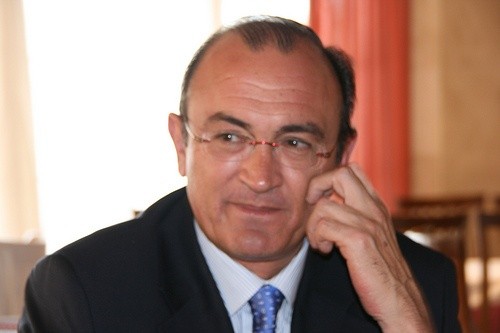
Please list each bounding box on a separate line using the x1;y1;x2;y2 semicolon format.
183;121;338;171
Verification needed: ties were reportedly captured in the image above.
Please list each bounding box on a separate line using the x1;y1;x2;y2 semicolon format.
250;284;283;332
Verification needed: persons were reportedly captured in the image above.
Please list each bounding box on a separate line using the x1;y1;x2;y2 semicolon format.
6;15;466;332
